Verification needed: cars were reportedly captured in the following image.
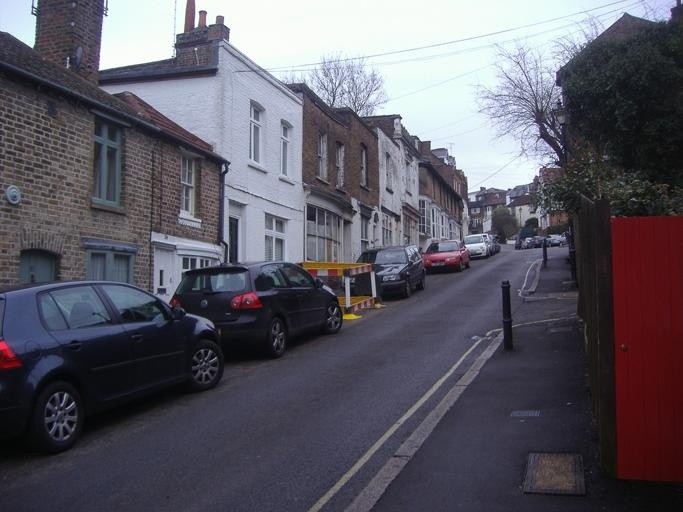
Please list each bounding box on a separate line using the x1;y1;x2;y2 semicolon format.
356;245;427;300
1;281;226;457
170;262;343;359
463;233;501;260
522;233;567;249
424;240;470;272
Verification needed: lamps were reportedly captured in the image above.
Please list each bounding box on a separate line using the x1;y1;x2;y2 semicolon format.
371;239;379;244
